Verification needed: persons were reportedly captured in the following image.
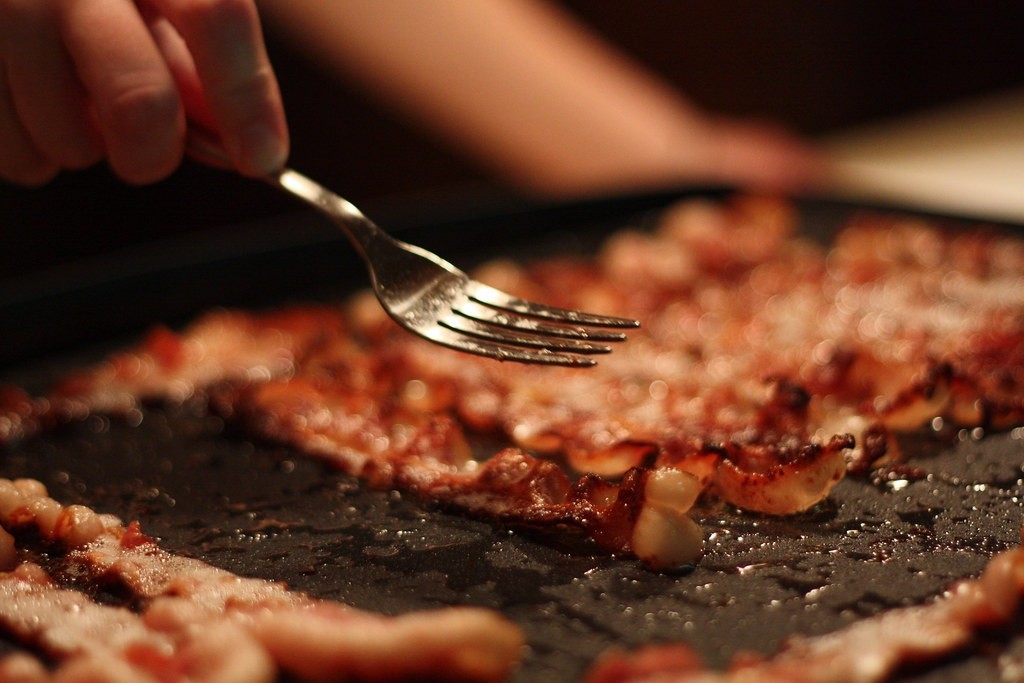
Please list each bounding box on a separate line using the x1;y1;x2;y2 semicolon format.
0;0;866;200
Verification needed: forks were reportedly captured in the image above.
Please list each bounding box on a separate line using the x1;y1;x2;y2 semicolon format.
179;120;640;368
